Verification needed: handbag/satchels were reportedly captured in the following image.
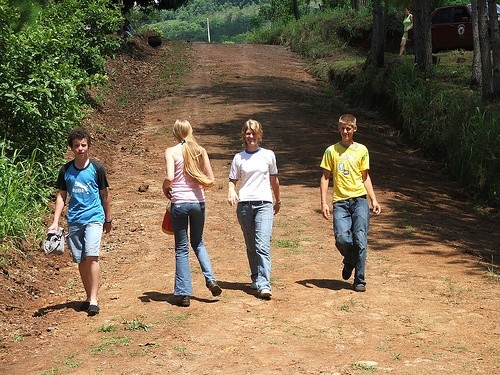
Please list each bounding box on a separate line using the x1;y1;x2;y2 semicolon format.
162;200;174;234
408;15;413;39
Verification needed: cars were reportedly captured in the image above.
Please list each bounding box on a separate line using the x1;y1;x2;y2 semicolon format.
405;1;500;53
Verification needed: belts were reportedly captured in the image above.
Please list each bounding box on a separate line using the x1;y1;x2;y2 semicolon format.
239;201;272;205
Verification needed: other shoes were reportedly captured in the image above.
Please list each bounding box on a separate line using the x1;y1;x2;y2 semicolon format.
342;264;353;280
251;281;258;290
76;301;90;311
167;293;190;306
87;305;100;315
259;289;272;297
208;280;222;297
353;284;366;291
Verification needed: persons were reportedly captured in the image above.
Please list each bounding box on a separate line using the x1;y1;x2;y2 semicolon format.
163;119;222;307
398;6;414;57
320;115;382;292
47;128;113;315
228;119;281;299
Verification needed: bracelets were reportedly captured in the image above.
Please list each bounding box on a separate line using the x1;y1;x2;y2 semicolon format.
163;184;168;192
276;200;281;204
105;219;112;223
